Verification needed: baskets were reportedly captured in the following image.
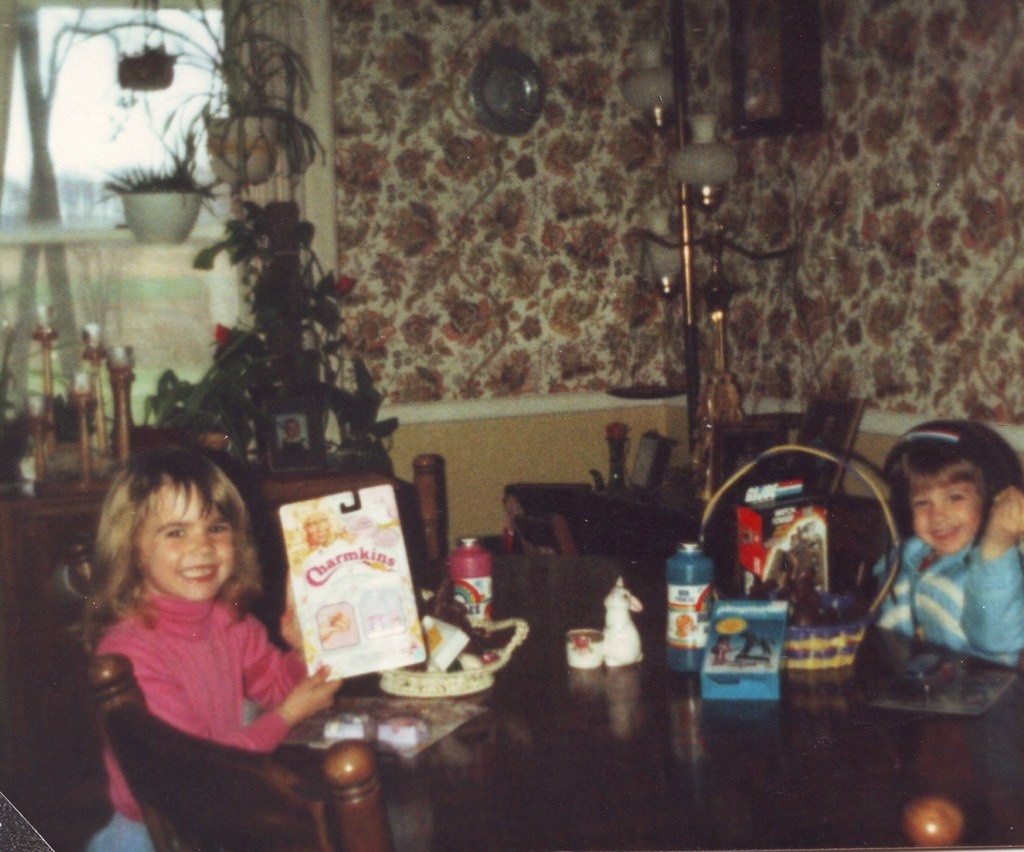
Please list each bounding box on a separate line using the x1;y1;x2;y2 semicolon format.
700;441;902;670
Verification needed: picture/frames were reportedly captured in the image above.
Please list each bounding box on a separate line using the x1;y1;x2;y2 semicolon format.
707;418;788;504
253;386;325;473
794;396;870;498
729;0;823;141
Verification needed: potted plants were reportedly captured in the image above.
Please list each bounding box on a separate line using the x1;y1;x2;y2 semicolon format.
48;0;217;90
181;0;331;196
143;200;400;471
107;136;219;245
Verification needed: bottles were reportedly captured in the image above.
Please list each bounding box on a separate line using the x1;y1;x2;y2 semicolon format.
665;541;715;675
448;536;494;638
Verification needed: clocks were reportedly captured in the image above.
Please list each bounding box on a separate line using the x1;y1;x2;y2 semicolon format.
468;51;546;132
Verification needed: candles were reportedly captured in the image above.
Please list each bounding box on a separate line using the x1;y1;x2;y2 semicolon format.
35;304;54;332
107;345;131;369
78;323;109;352
73;374;92;396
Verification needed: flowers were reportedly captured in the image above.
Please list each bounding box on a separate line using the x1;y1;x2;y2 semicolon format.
606;420;629;440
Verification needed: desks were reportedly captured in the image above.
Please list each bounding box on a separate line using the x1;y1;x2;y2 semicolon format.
282;625;1024;850
505;483;679;555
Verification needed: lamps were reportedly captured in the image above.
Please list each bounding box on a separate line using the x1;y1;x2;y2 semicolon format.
627;114;804;508
604;0;699;497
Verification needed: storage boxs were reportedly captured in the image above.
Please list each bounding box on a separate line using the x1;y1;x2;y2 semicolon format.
737;505;829;594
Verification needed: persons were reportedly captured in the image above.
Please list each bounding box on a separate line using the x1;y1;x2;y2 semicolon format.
873;419;1024;668
75;447;344;852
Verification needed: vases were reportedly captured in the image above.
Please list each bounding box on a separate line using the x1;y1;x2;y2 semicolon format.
604;438;630;495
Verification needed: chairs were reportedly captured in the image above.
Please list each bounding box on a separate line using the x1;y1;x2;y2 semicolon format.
89;652;395;852
236;451;446;594
